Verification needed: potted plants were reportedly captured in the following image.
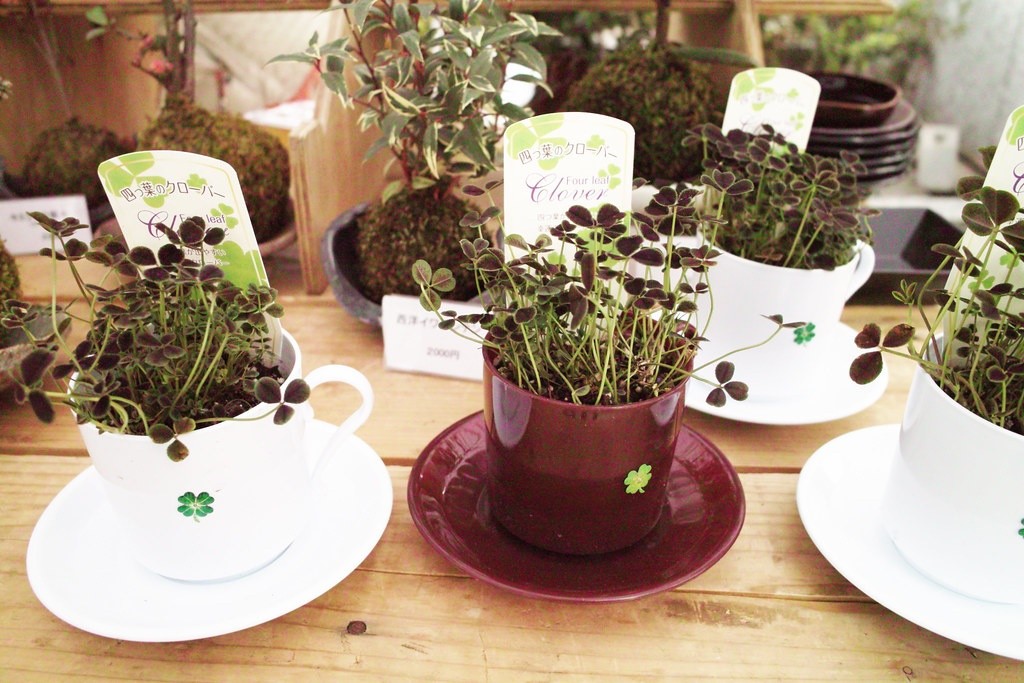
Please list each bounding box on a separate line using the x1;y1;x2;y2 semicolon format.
77;1;298;258
0;208;373;585
414;168;805;559
0;239;73;391
266;0;565;329
680;123;874;381
849;172;1024;597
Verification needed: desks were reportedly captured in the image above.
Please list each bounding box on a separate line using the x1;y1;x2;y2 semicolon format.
4;299;1024;682
855;176;982;275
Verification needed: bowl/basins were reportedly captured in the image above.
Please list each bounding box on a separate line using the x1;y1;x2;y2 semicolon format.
805;72;901;128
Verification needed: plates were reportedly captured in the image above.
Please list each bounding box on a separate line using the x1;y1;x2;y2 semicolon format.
808;102;923;180
